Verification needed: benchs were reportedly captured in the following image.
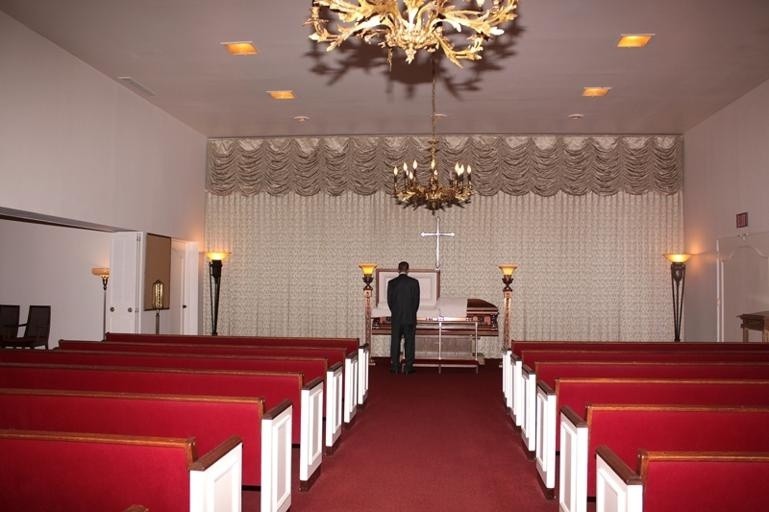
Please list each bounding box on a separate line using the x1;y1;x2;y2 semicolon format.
1;332;369;512
500;341;769;512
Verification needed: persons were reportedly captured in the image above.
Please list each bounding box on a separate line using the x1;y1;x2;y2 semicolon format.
387;261;420;375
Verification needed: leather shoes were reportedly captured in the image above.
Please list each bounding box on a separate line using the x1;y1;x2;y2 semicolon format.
390;367;416;374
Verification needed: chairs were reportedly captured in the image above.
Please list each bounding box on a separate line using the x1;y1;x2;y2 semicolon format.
0;303;56;349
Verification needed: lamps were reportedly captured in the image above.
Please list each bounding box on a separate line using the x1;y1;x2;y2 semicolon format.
93;266;113;344
663;249;691;343
497;264;516;371
392;53;472;212
359;264;380;368
204;247;232;336
303;1;518;72
